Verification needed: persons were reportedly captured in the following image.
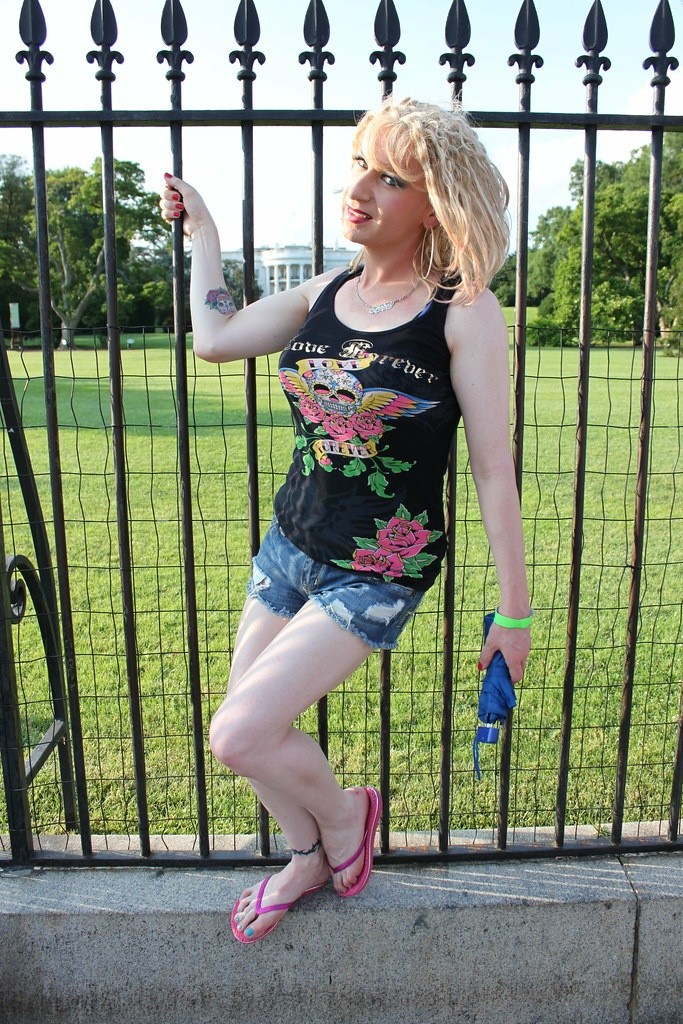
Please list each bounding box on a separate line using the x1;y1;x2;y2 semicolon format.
159;95;535;945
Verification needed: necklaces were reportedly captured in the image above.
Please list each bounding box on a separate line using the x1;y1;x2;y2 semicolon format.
355;274;426;313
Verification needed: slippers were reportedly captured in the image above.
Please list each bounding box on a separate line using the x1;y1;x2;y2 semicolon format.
326;786;382;897
231;871;329;942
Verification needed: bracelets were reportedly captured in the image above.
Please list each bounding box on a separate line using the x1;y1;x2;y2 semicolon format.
494;605;534;629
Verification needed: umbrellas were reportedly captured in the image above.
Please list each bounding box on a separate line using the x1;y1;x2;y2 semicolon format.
472;612;517;780
